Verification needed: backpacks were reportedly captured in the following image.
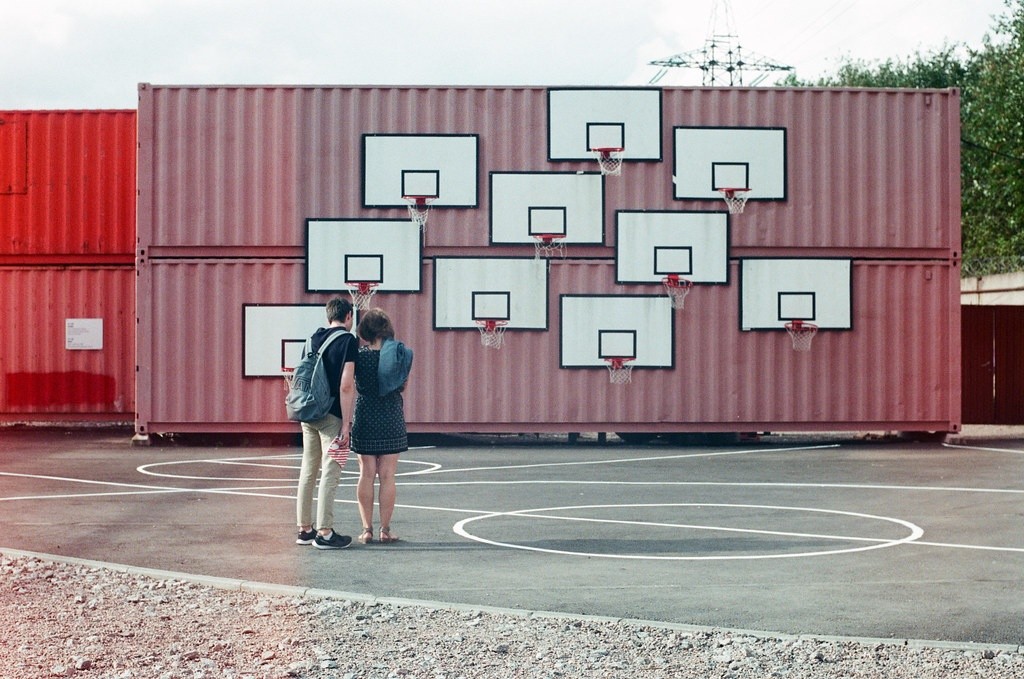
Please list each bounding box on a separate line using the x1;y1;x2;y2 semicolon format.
285;330;352;425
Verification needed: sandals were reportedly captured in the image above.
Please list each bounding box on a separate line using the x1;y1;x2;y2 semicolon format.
356;525;373;544
376;527;401;543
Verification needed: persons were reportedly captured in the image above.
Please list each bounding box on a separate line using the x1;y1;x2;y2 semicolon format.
284;296;357;550
350;308;409;544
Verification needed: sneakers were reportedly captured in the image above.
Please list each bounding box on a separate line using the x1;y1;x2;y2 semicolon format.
295;523;319;546
312;527;354;550
